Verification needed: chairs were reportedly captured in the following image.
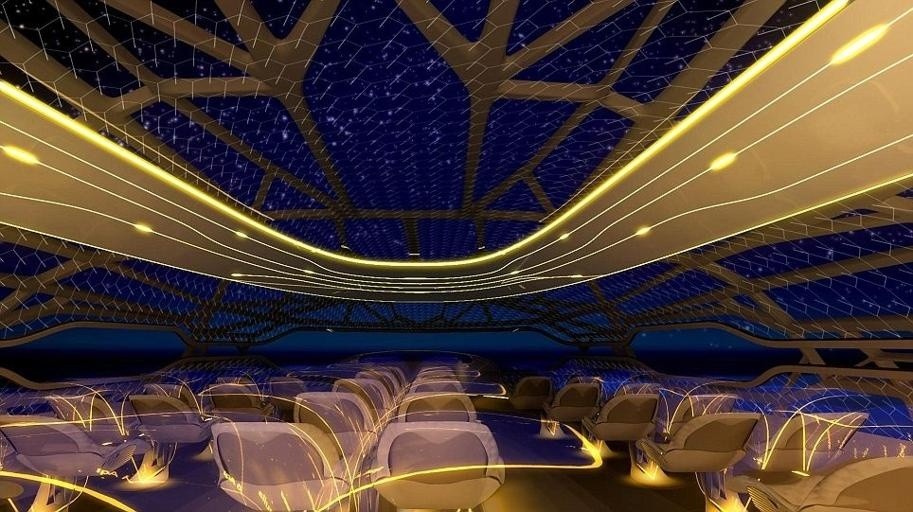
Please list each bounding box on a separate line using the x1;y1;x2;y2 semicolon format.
0;365;913;512
